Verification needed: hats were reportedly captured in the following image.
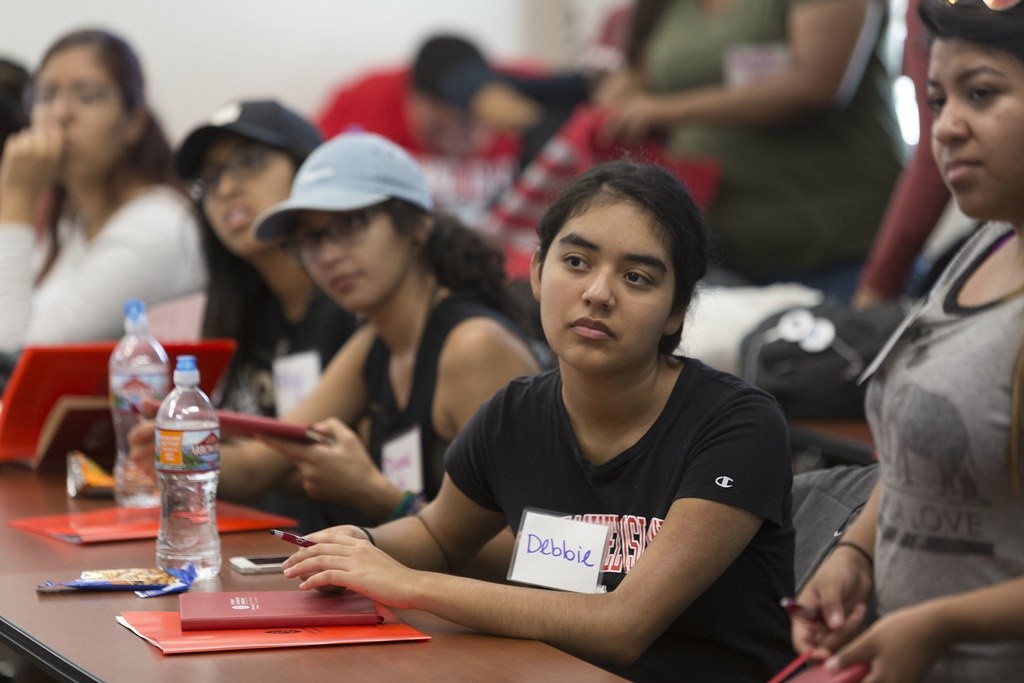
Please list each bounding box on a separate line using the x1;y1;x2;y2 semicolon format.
412;37;495;110
248;132;433;248
164;101;324;186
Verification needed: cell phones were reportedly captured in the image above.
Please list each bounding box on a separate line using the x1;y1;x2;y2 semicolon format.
228;554;290;576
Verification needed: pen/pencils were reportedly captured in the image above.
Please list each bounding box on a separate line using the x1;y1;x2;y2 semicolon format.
269;530;316;548
780;598;824;623
129;403;146;425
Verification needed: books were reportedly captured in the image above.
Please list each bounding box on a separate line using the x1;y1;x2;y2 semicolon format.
178;590;383;629
182;406;338;447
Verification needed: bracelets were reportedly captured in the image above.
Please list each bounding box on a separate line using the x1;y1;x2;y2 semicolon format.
358;525;376;546
379;491;414;526
834;542;873;564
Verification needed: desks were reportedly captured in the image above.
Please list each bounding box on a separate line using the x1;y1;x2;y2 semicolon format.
0;329;883;683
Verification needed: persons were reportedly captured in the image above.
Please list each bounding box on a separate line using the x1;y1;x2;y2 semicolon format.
594;0;909;277
132;131;547;580
321;32;546;278
791;0;1024;683
0;30;367;518
280;160;795;683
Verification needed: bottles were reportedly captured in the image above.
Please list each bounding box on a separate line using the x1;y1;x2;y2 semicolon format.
108;297;171;511
152;354;224;582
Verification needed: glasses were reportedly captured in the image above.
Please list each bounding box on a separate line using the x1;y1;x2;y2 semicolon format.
188;143;269;200
290;201;395;256
